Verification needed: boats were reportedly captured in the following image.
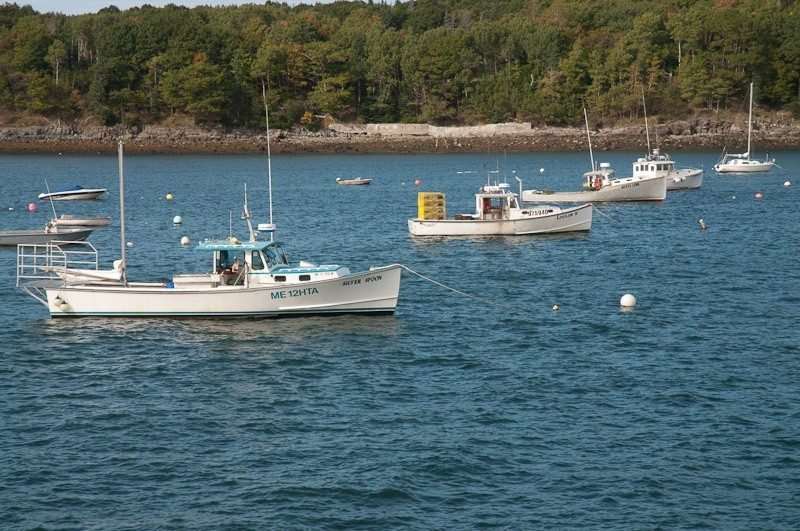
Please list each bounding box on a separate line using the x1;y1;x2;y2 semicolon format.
48;214;112;226
37;185;107;202
0;222;95;248
628;88;705;190
407;170;596;236
513;107;668;202
14;86;404;318
335;176;373;187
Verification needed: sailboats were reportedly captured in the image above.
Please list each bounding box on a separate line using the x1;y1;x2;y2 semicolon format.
713;81;778;174
